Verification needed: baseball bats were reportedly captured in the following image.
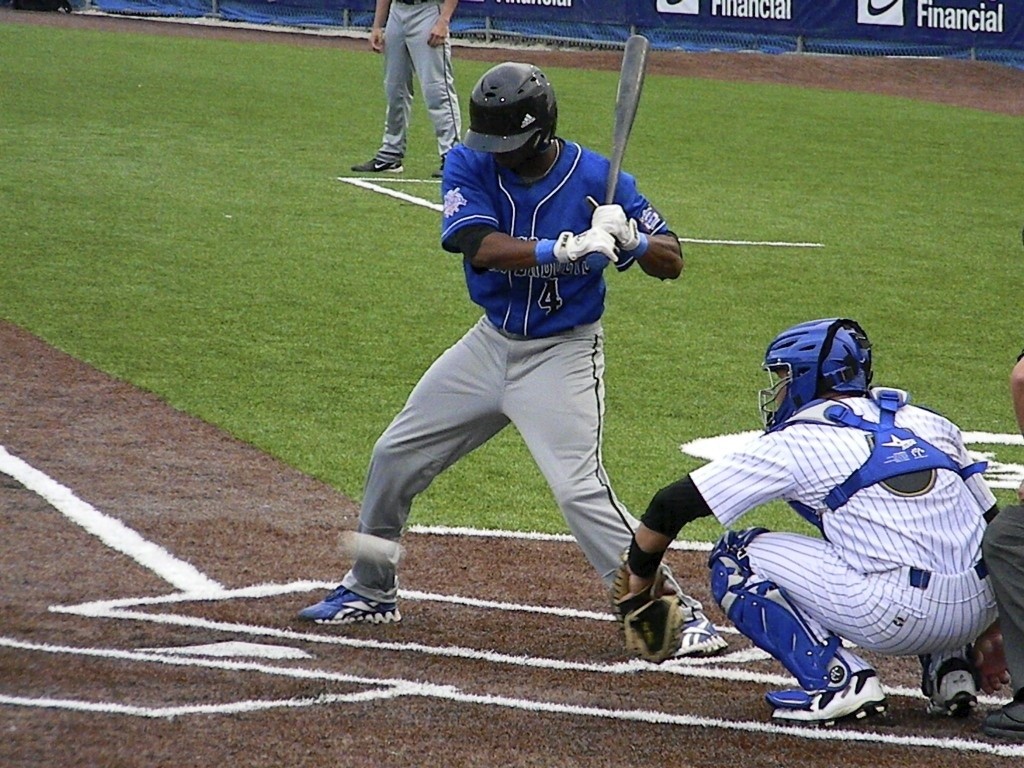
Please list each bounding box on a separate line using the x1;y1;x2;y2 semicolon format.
585;36;651;271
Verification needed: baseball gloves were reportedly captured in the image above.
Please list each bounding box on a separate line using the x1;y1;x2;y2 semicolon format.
607;545;685;664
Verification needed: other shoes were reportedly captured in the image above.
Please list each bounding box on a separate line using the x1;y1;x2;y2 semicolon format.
982;696;1024;743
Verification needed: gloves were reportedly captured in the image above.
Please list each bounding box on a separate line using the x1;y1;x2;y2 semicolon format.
551;226;621;264
590;203;641;252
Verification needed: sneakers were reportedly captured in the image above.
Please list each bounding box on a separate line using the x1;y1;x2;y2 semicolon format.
297;583;403;628
766;667;889;731
924;656;981;720
672;611;729;661
351;157;404;174
431;153;448;177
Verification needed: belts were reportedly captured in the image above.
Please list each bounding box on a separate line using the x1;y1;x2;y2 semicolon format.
396;0;429;6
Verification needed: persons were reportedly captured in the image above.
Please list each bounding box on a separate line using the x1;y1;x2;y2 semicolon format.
299;61;728;656
982;349;1024;737
351;0;461;176
611;316;1012;723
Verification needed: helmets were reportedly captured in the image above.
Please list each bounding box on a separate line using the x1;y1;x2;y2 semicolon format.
756;316;874;432
460;61;558;155
